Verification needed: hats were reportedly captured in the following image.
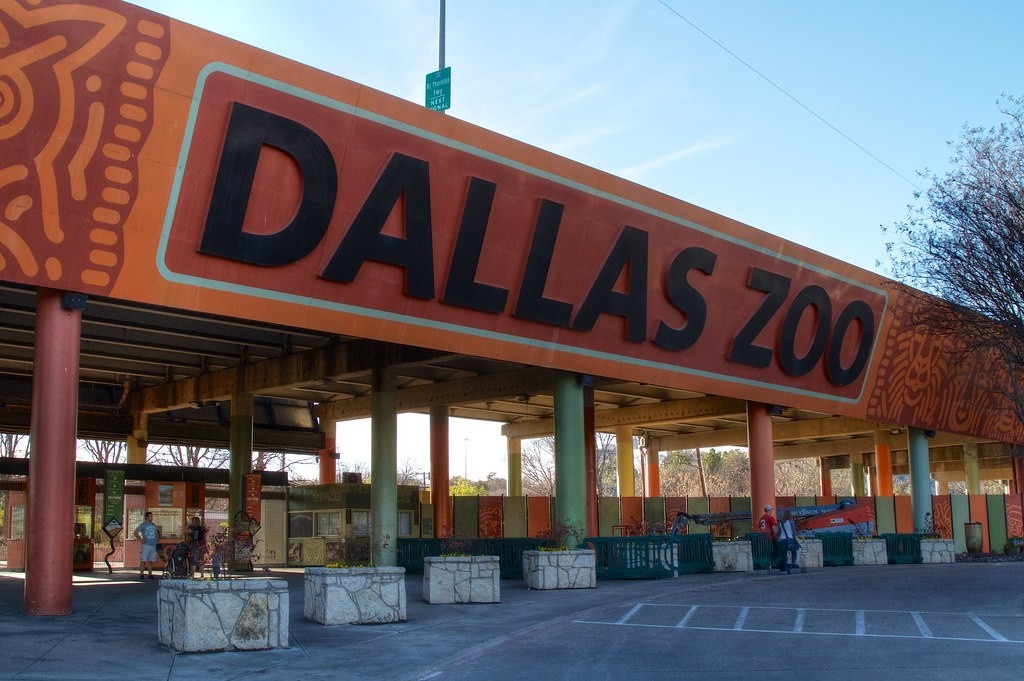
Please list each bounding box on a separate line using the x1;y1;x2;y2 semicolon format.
765;505;773;510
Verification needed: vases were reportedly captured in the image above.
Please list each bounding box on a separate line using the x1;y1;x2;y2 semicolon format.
614;543;678;578
423;556;500;605
521;548;597;590
920;538;955;564
787;539;823;568
157;577;289;652
711;541;754;572
852;538;888;565
304;566;407;625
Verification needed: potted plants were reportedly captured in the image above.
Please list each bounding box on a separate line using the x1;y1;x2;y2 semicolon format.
1009;536;1024;545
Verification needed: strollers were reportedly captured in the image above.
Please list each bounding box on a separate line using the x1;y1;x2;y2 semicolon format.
161;542;192;580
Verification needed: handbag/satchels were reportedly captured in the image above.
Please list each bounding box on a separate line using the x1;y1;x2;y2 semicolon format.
786;534;799;551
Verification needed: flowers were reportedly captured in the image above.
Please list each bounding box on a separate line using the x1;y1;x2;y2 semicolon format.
847;516;882;540
916;512;946;539
708;520;743;542
162;522;271;582
794;520;810;540
438;528;484;557
323;524;381;568
531;517;585;551
619;511;667;543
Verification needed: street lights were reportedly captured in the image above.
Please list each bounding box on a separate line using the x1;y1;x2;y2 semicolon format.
415;472;430;487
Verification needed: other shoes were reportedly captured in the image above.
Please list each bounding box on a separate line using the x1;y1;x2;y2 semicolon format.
200;576;204;580
189;576;194;580
140;575;144;580
766;561;772;572
148;575;154;579
790;564;799;569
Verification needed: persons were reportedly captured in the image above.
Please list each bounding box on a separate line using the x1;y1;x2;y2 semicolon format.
778;510;802;574
208;544;223;580
758;504;777;573
134;511;159;580
181;516;207;580
